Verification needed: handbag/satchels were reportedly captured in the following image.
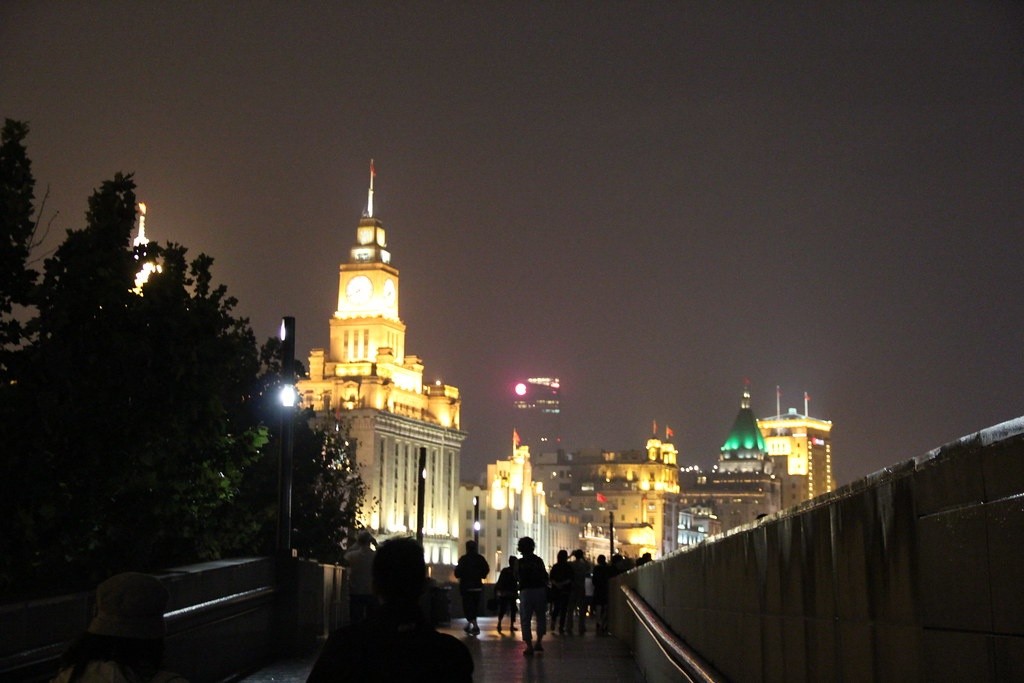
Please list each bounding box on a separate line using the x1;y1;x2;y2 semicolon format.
349;570;372;595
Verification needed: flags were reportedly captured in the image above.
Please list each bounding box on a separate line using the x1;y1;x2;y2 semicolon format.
652;422;658;433
597;493;607;502
512;432;520;446
666;427;674;440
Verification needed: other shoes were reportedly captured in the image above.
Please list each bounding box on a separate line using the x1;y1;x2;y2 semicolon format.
551;625;555;631
523;649;534;657
559;627;564;633
533;645;543;651
497;626;502;632
510;627;517;631
463;626;471;633
470;627;480;635
579;630;586;636
567;630;572;635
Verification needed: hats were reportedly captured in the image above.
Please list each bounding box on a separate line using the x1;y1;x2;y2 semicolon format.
88;573;168;640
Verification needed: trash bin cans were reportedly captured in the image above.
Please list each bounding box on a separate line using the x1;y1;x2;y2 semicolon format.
298;559;350;645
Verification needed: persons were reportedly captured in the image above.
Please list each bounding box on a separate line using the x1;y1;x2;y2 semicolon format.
549;549;652;637
454;540;489;636
515;537;549;657
495;556;518;631
48;571;192;683
305;536;475;683
344;531;378;618
